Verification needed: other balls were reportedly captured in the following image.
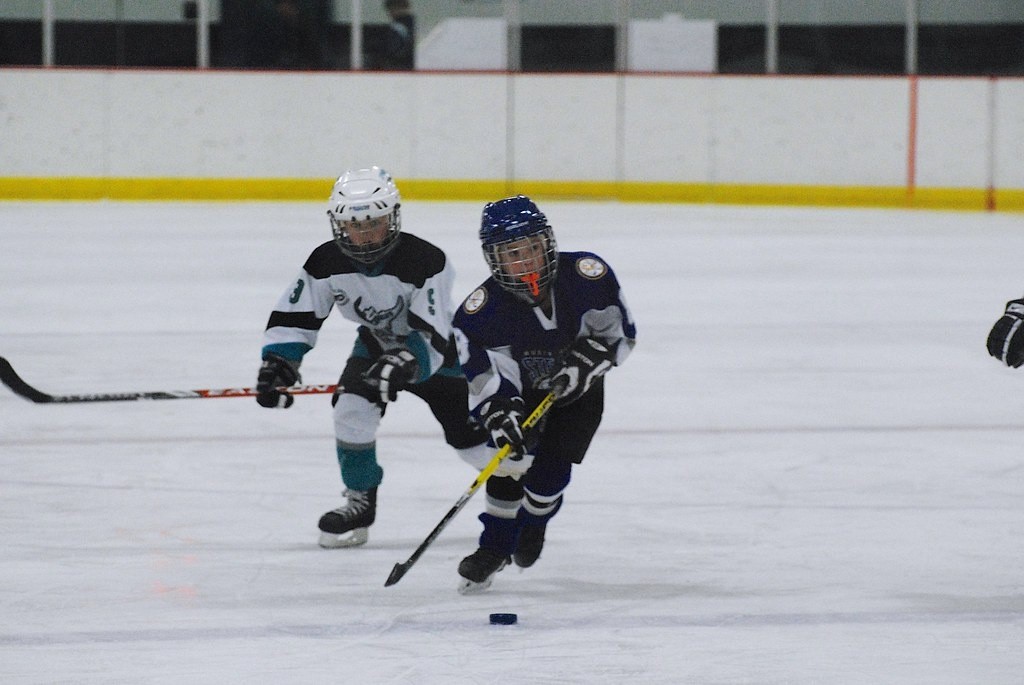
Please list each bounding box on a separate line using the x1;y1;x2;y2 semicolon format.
489;613;518;625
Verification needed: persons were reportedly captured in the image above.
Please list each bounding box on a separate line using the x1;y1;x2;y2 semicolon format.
258;166;496;548
450;194;638;593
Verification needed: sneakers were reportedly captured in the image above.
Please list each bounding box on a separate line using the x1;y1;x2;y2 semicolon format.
513;494;563;573
318;488;377;549
458;512;528;594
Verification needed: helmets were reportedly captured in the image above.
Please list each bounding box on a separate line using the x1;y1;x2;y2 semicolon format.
327;166;401;263
479;193;559;294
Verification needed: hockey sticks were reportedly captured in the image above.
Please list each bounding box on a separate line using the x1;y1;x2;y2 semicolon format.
0;351;358;406
385;385;564;588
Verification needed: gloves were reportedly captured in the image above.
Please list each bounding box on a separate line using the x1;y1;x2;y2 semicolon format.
255;351;302;408
366;348;420;402
548;336;619;407
987;297;1024;368
479;396;527;461
